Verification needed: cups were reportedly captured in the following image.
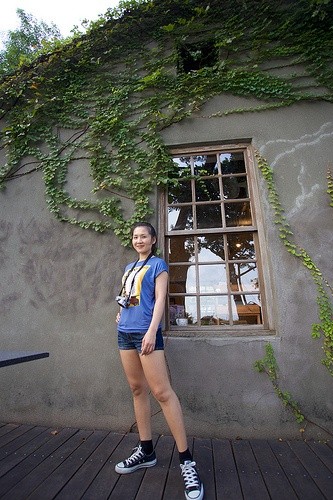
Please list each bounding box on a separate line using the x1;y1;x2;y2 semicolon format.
176;318;188;326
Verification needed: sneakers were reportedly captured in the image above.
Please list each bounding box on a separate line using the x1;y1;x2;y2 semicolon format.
115;445;157;473
180;461;204;500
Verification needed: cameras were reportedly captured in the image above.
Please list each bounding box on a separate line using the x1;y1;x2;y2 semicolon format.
115;296;130;308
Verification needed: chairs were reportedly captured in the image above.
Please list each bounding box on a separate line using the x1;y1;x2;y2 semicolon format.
217;284;261;326
